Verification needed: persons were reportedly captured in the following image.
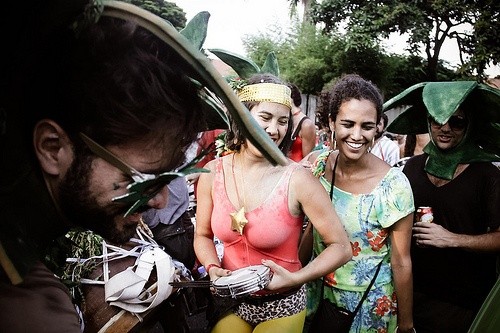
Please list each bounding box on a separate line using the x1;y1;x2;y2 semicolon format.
286;83;315;164
297;74;418;333
194;73;353;333
399;81;500;333
365;111;400;168
1;1;288;333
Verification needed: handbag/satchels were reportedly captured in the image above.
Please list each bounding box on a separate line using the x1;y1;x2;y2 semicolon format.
302;299;355;333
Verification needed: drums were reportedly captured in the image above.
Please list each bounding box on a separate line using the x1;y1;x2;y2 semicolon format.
212;265;273;299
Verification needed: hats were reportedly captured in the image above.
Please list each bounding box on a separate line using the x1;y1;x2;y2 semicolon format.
381;81;500;180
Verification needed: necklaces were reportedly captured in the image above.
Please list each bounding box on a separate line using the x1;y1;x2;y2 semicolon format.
229;152;271;236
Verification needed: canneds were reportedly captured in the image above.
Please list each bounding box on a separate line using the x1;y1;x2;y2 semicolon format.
416;207;434;247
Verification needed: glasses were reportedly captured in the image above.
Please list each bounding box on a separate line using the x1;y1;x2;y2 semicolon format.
62;125;216;220
426;115;467;129
